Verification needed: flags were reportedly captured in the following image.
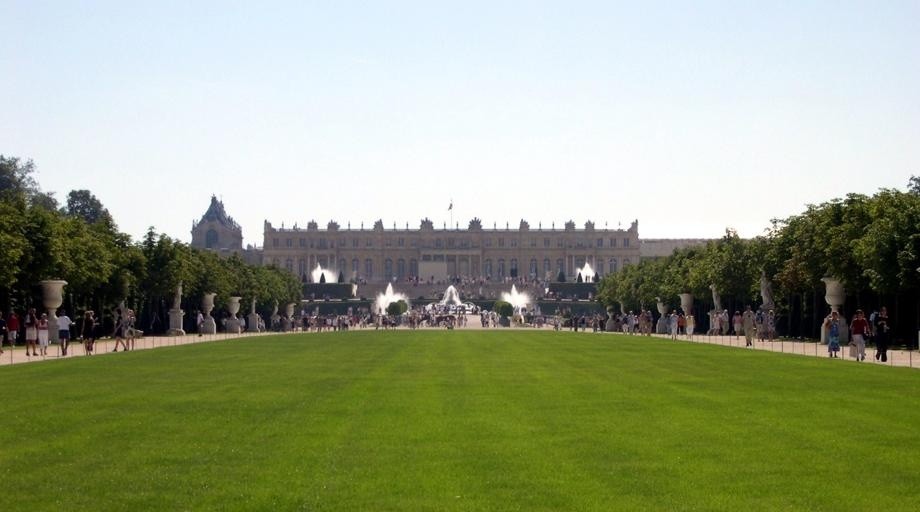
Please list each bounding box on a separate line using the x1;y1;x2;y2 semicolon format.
447;203;453;211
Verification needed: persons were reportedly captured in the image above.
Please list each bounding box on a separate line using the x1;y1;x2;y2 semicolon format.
0;312;7;354
850;309;871;362
825;312;840;358
6;311;20;348
473;305;500;328
868;310;878;336
112;309;126;352
553;309;653;337
513;306;543;328
196;310;205;337
223;312;354;333
712;304;775;346
24;308;38;356
56;309;72;356
36;313;49;356
359;304;467;331
124;310;136;351
874;306;890;362
90;310;100;354
81;311;94;357
670;309;695;341
